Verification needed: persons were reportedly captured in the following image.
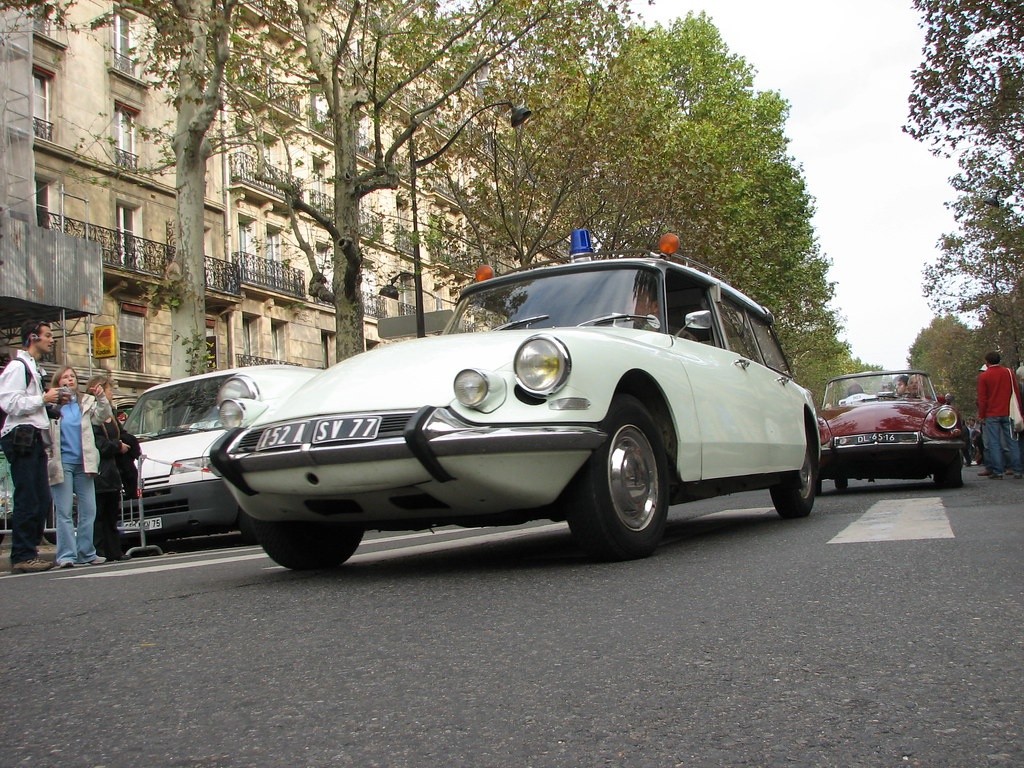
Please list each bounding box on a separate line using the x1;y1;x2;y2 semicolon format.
907;374;923;396
624;280;658;329
976;351;1024;480
46;365;113;568
847;383;863;396
954;363;1024;476
895;376;908;395
0;320;70;574
85;376;141;563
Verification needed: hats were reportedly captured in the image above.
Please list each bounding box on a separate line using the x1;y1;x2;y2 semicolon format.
979;364;988;371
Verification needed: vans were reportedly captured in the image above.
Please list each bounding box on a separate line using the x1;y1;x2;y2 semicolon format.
117;364;326;558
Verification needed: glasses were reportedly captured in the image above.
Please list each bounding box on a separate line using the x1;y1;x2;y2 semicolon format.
34;321;46;332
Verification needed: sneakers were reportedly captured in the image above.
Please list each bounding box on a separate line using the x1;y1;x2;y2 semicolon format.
12;559;55;574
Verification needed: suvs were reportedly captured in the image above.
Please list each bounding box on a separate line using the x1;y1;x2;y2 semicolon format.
202;228;821;572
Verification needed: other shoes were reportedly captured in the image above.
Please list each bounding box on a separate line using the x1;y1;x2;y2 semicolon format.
1006;467;1014;475
988;474;1005;480
89;556;106;565
117;554;132;560
977;469;992;476
60;561;73;568
1015;472;1022;479
971;461;977;465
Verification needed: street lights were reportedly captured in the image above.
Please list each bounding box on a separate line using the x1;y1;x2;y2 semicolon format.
378;99;532;338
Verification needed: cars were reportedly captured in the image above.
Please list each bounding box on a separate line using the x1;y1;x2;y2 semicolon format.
816;371;965;495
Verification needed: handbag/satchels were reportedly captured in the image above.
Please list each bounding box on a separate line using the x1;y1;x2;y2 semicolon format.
1009;392;1024;441
